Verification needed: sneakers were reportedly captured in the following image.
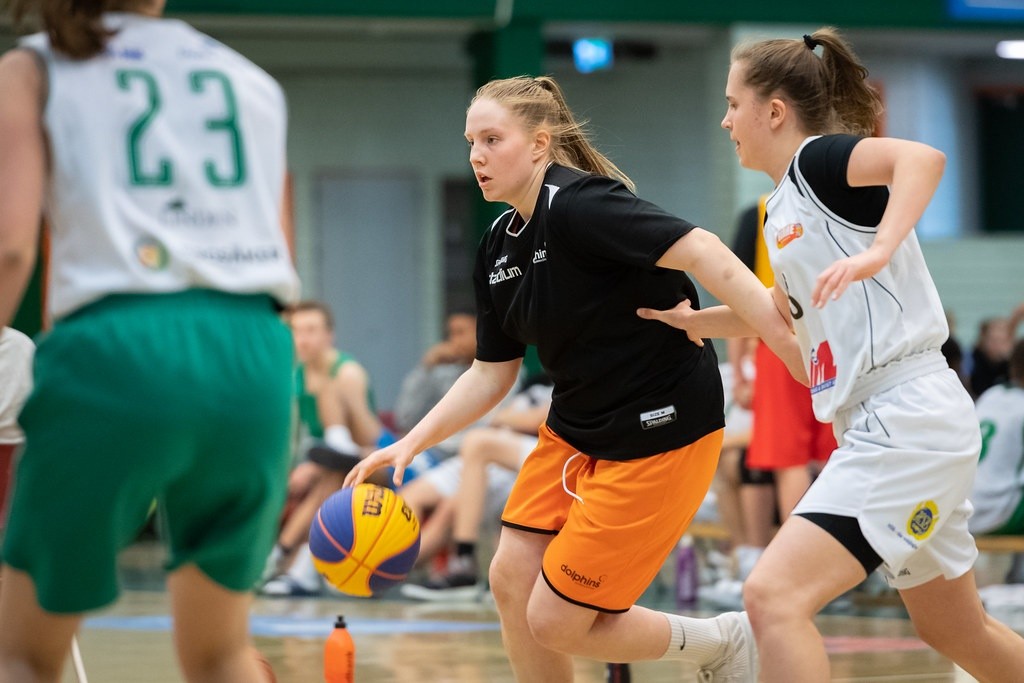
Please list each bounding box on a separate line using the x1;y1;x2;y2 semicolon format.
695;609;757;683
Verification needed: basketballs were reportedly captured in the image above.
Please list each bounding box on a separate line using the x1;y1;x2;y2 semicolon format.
308;483;421;598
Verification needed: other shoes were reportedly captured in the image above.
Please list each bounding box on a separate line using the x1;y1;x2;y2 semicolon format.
256;570;297;598
699;548;760;611
400;556;479;601
287;566;322;595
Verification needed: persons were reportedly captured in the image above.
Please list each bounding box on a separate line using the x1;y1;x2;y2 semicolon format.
694;186;1022;612
636;26;1023;683
257;302;554;596
0;0;307;683
341;76;807;683
